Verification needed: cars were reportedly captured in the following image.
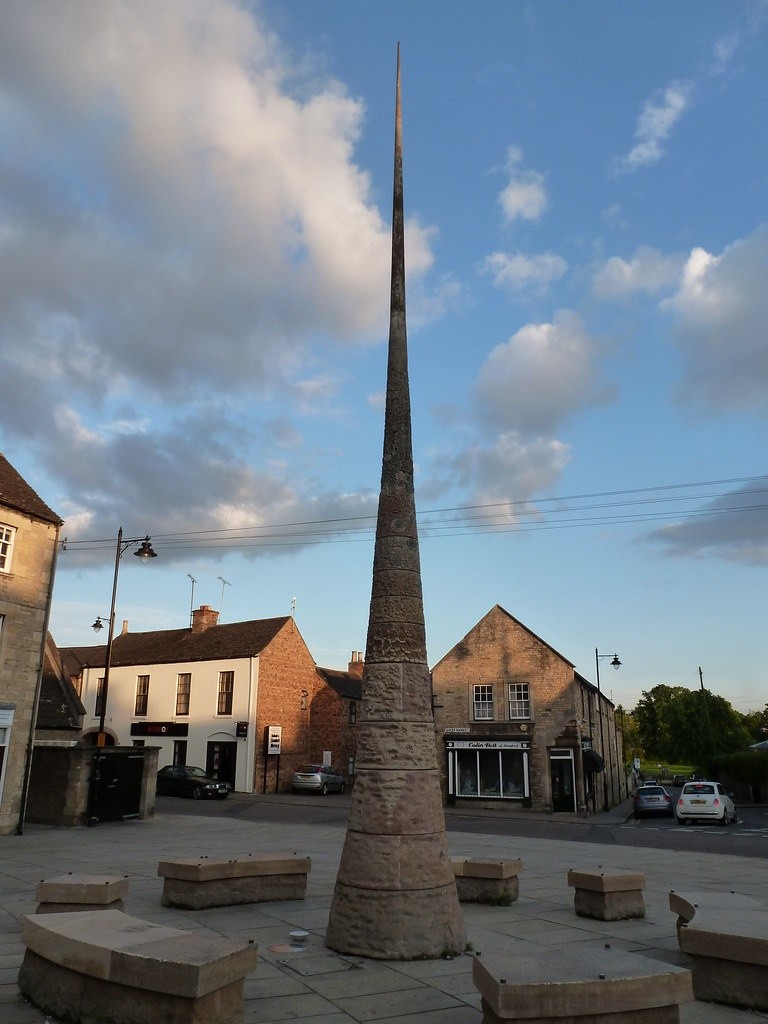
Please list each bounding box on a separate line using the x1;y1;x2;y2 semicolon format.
630;784;675;819
155;765;232;801
671;774;687;787
674;780;737;827
291;763;346;796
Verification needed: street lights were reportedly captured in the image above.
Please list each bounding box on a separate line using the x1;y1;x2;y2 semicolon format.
83;526;159;827
594;647;623;812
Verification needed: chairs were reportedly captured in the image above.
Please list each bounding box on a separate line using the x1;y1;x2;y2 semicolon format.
704;788;712;793
687;788;695;793
